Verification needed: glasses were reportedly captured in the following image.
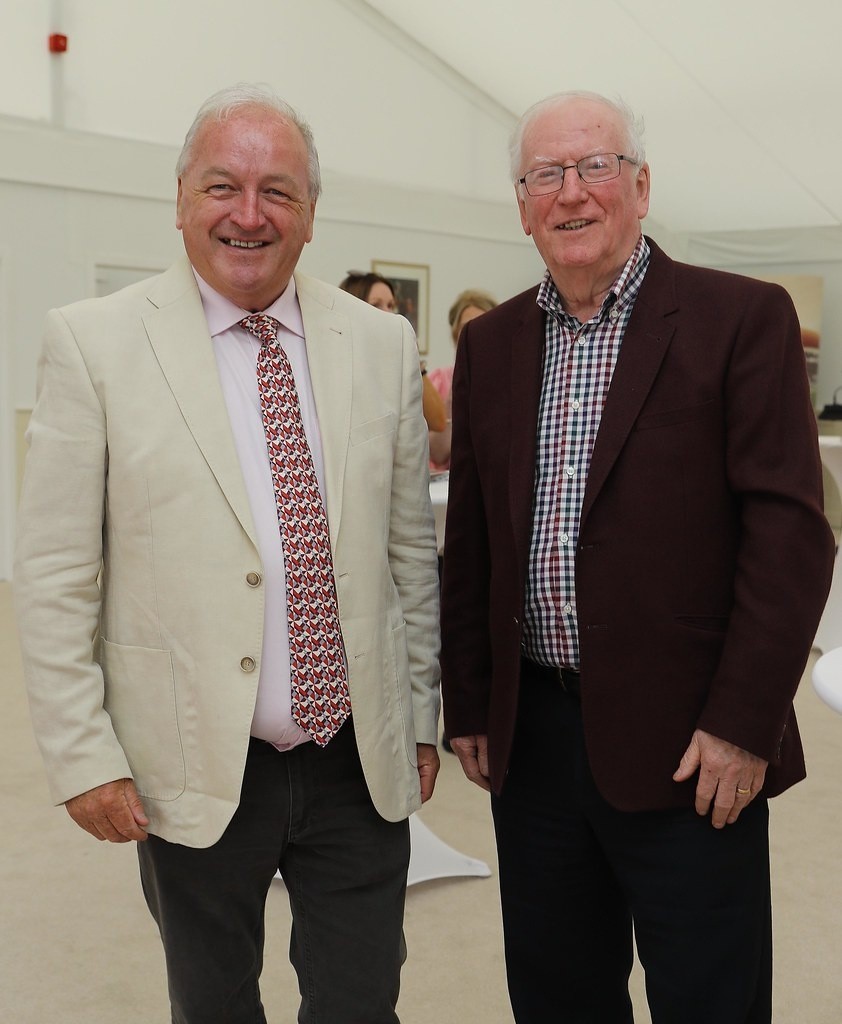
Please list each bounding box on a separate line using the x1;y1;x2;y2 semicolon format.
517;151;640;196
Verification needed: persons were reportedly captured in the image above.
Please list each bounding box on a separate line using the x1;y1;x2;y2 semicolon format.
438;87;836;1024
333;267;499;472
16;84;442;1024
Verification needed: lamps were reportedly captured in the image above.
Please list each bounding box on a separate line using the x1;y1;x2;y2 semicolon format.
818;386;842;421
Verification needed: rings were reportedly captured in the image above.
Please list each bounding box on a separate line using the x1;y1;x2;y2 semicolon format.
737;788;751;795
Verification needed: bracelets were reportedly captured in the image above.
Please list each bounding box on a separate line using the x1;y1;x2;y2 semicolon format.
421;369;427;377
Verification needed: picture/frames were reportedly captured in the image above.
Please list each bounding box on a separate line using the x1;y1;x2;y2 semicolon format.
371;260;430;355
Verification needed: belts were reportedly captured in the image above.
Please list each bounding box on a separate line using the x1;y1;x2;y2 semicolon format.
522;660;583;693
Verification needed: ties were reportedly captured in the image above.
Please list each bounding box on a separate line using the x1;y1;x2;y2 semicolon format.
238;313;353;748
